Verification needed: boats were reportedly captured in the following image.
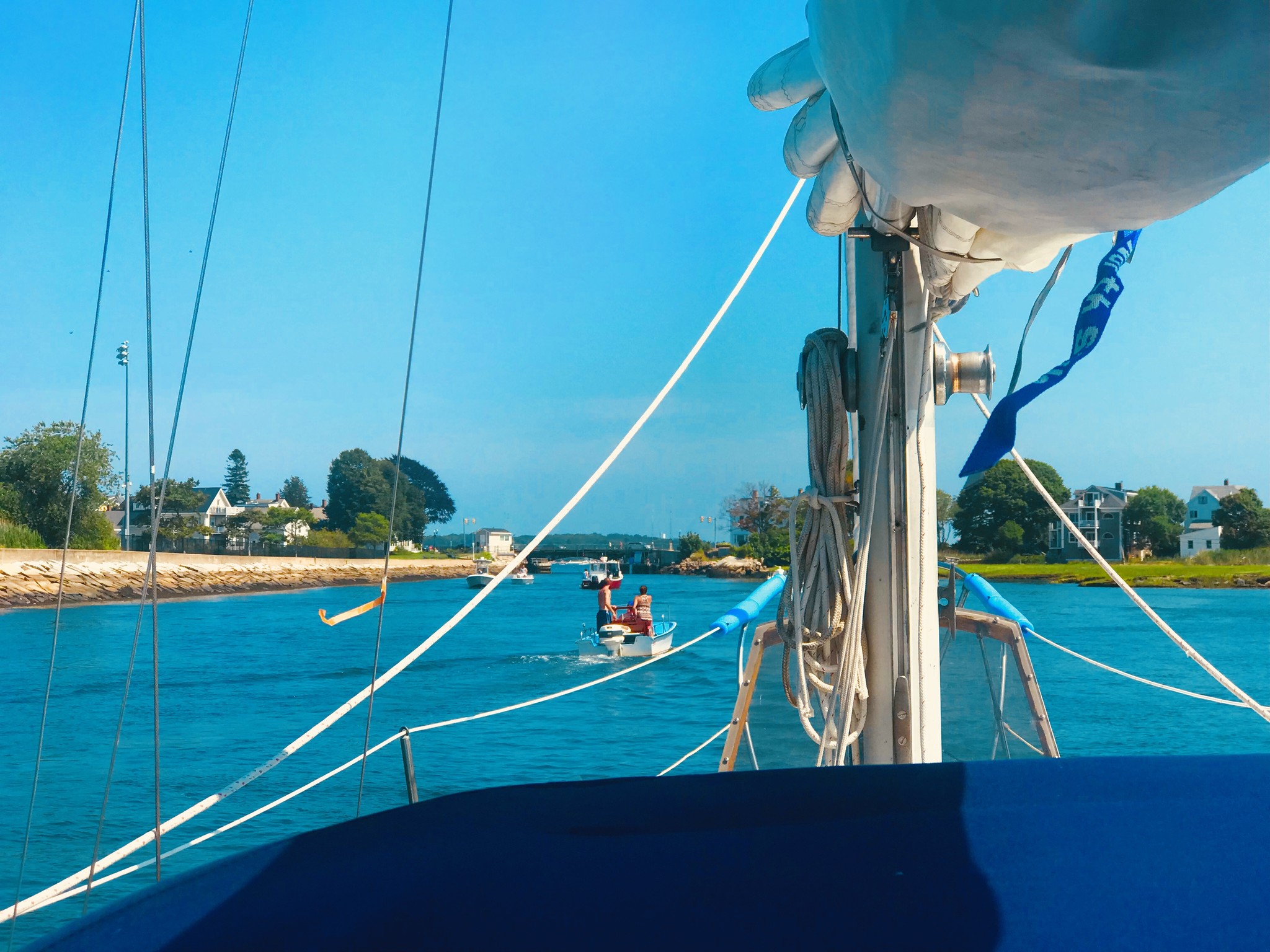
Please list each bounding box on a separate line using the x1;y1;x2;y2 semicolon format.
511;575;534;585
574;600;677;658
580;551;624;590
465;542;496;589
528;557;552;571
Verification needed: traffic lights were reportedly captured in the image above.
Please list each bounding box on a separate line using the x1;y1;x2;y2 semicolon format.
701;516;703;522
708;517;711;523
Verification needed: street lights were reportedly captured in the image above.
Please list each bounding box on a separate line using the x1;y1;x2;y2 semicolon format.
434;529;438;548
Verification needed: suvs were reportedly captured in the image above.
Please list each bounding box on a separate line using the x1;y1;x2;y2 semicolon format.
627;542;652;551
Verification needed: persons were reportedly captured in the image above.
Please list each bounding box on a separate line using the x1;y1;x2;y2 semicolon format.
596;577;617;634
582;569;588;580
633;586;653;637
627;601;636;622
651;540;654;550
619;541;623;549
520;565;528;573
609;542;612;549
